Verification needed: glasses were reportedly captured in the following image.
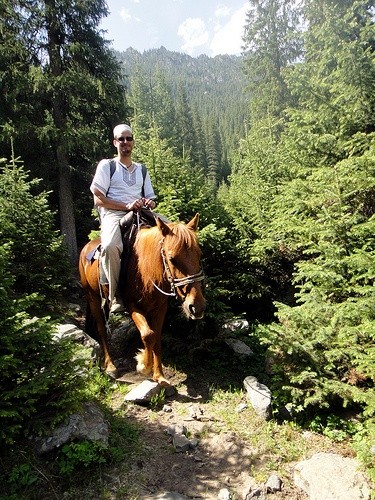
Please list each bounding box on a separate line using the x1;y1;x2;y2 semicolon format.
112;137;133;141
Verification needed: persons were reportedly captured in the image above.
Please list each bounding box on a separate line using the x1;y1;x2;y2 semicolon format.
89;124;158;314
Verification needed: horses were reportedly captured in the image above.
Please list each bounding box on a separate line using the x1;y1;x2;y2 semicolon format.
78;212;205;389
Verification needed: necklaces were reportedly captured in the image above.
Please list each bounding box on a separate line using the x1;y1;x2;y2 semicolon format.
127;164;132;168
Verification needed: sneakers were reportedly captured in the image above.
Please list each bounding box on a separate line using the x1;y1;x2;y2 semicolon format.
110;300;123;313
